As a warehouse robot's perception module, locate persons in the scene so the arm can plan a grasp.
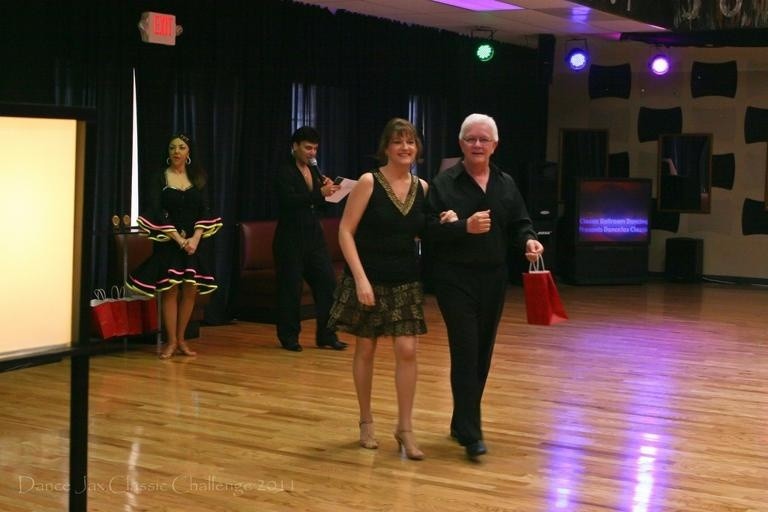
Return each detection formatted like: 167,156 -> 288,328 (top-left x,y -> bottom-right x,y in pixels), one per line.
125,134 -> 226,359
421,114 -> 545,457
326,118 -> 460,460
272,125 -> 350,352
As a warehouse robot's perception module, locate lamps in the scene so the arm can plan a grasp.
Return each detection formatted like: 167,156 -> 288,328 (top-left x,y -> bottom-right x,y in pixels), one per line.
565,38 -> 590,72
472,31 -> 497,63
650,47 -> 671,76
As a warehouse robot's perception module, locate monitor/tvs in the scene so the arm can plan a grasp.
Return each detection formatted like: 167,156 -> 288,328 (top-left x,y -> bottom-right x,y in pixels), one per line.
561,176 -> 652,245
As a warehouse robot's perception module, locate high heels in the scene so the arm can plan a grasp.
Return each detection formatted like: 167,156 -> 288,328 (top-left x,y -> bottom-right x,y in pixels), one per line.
360,415 -> 378,449
395,429 -> 426,459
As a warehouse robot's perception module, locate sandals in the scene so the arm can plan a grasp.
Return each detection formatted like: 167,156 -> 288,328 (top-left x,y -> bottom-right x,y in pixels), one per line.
160,341 -> 197,359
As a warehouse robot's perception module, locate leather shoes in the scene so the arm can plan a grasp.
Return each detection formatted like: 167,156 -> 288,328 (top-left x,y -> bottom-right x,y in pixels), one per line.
282,341 -> 303,352
317,339 -> 349,349
451,426 -> 486,456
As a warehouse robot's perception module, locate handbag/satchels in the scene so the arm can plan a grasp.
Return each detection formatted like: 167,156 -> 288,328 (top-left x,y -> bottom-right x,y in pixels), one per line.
89,285 -> 158,340
522,252 -> 569,326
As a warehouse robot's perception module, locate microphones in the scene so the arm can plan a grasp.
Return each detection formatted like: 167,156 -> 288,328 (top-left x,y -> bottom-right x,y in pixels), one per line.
308,157 -> 324,187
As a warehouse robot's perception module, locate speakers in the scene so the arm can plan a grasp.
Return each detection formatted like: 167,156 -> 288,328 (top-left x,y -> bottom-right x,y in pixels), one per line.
538,34 -> 555,65
666,237 -> 703,283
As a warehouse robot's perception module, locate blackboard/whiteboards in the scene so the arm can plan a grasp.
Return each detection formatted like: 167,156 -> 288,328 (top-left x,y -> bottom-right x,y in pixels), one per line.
0,112 -> 82,365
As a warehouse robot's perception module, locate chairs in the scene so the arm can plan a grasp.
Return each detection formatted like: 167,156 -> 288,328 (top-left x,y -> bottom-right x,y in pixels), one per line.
113,233 -> 212,347
234,217 -> 351,321
